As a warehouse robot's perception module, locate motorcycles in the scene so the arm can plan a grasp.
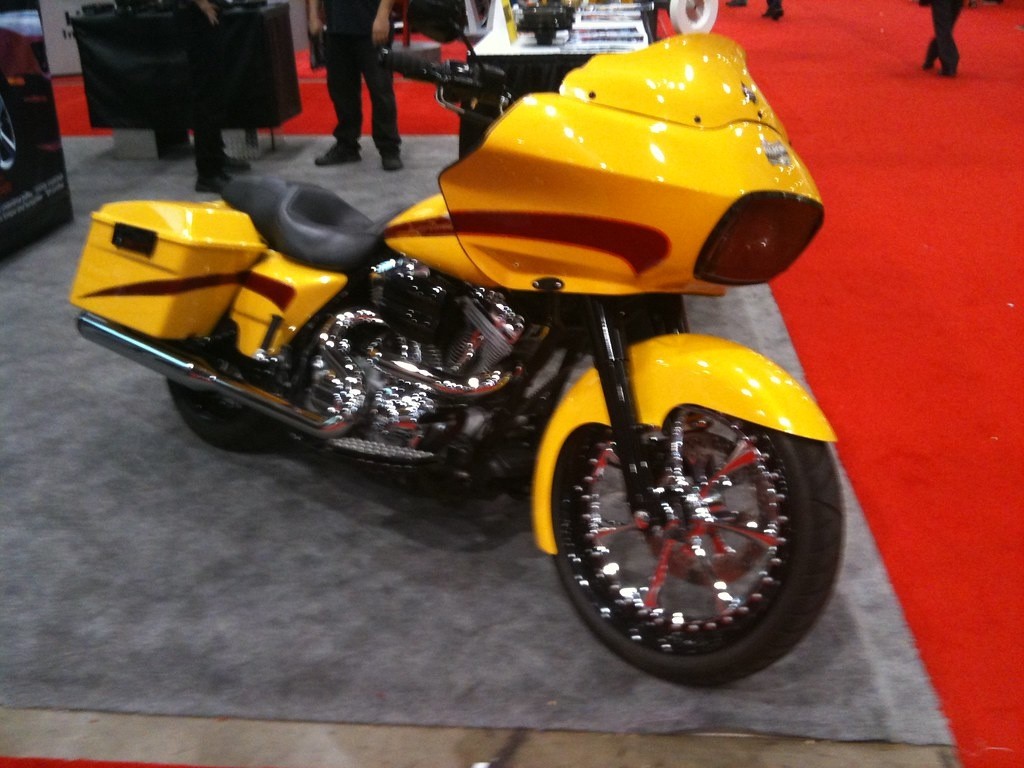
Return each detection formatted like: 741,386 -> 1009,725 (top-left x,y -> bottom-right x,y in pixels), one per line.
62,32 -> 851,691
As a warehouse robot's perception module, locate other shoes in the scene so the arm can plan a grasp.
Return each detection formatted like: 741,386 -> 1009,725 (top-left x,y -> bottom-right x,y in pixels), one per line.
195,172 -> 232,192
771,6 -> 782,19
922,50 -> 936,70
218,154 -> 249,171
380,151 -> 402,169
939,64 -> 956,75
315,146 -> 363,166
726,0 -> 746,6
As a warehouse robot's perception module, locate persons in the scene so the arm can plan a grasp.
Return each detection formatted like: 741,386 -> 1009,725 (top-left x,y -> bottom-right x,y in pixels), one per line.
155,0 -> 405,193
727,0 -> 784,20
919,0 -> 977,76
